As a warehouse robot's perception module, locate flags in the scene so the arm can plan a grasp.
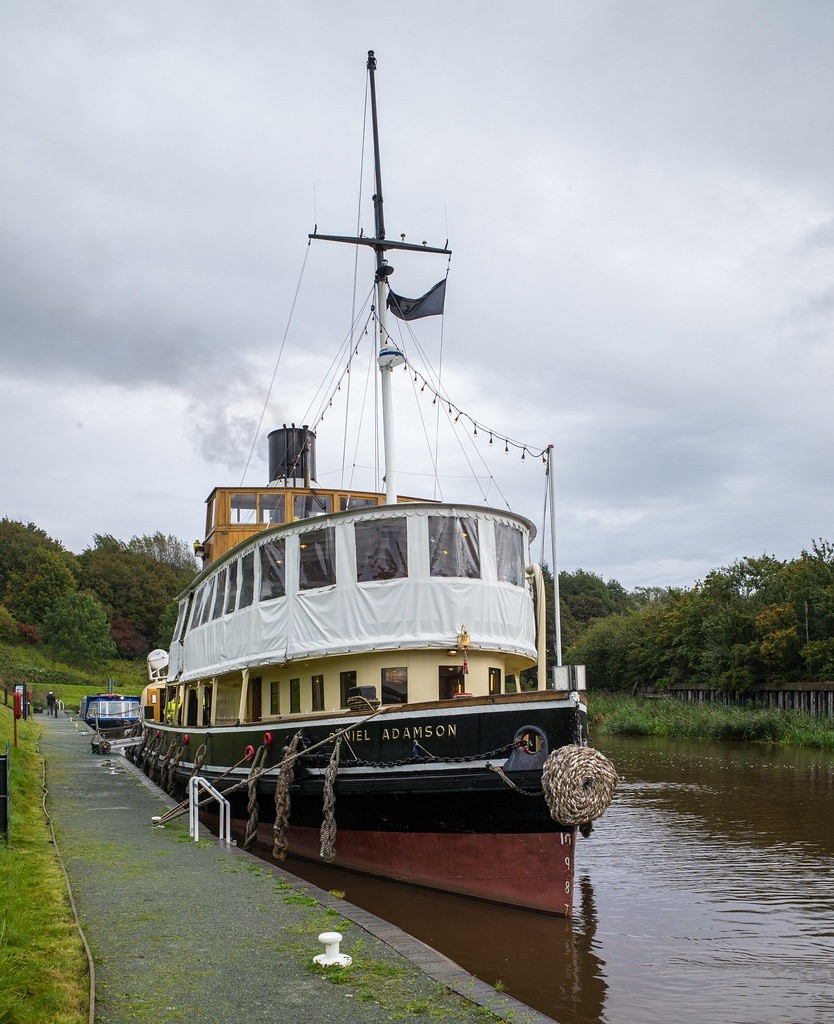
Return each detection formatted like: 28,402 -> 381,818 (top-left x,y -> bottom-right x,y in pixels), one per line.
386,279 -> 445,320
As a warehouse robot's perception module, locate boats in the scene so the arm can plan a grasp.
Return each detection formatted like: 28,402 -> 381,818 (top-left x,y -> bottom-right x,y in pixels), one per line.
76,677 -> 141,732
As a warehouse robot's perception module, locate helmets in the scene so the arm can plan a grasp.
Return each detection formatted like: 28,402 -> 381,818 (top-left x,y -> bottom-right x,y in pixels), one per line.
49,692 -> 53,694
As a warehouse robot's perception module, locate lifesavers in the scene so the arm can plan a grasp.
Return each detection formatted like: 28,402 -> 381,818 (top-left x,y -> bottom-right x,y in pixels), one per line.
245,744 -> 255,762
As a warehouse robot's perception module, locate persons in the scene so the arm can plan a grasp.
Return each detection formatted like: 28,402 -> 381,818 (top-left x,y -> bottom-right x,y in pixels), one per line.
162,693 -> 183,727
46,692 -> 55,715
178,689 -> 208,727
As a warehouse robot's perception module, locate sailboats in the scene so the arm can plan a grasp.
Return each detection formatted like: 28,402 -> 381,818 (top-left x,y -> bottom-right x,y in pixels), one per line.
124,45 -> 626,922
